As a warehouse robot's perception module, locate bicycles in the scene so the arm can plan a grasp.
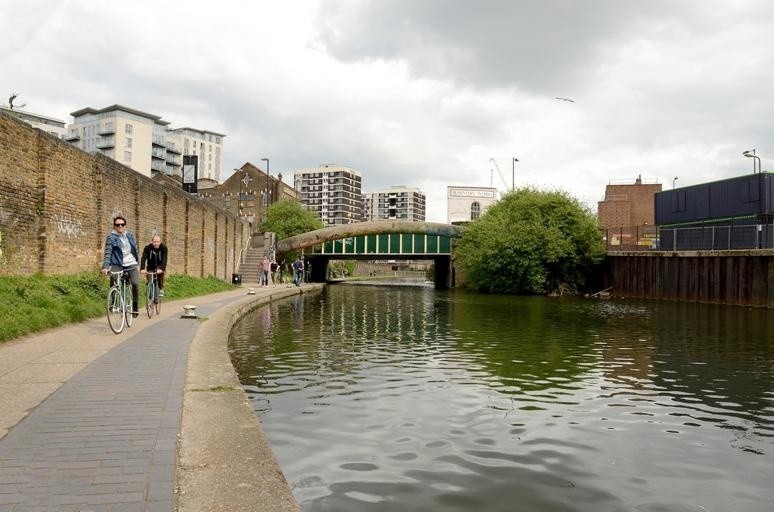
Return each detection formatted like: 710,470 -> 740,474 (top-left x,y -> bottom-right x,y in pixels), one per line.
100,267 -> 139,334
145,270 -> 164,319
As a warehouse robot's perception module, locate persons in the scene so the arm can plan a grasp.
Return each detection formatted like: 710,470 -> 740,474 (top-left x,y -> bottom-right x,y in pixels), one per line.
140,235 -> 168,297
260,255 -> 312,288
101,216 -> 139,318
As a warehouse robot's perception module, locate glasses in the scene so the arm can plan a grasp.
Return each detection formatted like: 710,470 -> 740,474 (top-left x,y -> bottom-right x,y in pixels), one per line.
114,223 -> 126,227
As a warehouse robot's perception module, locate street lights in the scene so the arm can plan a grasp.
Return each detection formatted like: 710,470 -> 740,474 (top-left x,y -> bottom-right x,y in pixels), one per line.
672,177 -> 679,189
513,157 -> 519,191
742,148 -> 762,175
233,168 -> 242,215
260,159 -> 269,206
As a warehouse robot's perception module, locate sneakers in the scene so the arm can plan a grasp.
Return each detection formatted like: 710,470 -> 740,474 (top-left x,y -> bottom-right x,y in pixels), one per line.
109,301 -> 117,312
132,306 -> 139,318
158,290 -> 164,297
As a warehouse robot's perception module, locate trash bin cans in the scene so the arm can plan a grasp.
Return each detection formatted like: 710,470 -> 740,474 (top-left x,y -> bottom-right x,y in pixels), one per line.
232,273 -> 241,284
